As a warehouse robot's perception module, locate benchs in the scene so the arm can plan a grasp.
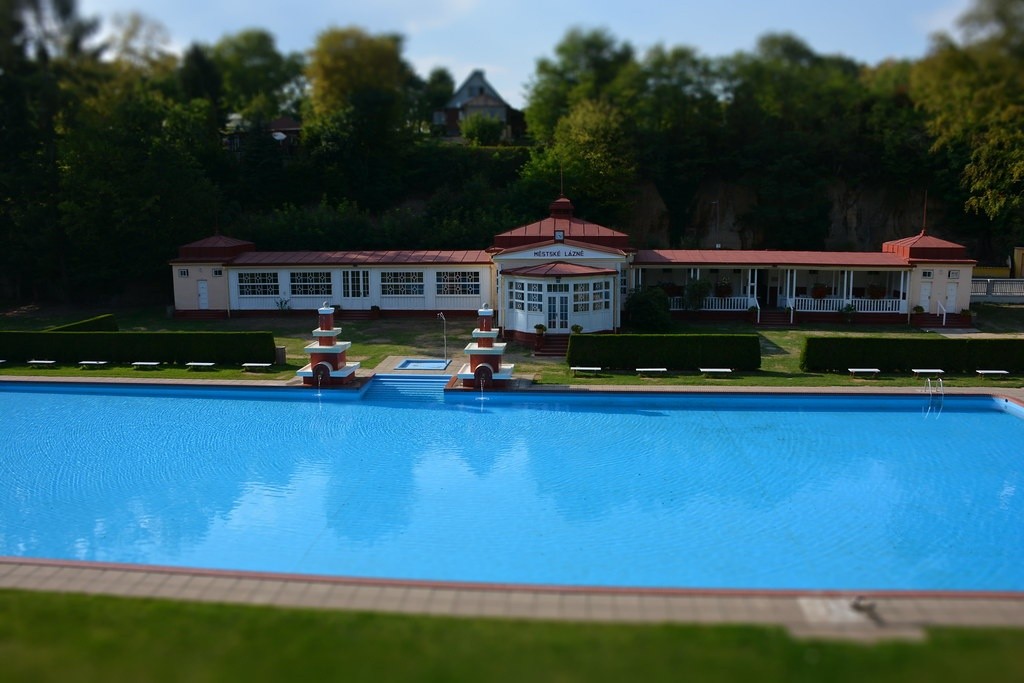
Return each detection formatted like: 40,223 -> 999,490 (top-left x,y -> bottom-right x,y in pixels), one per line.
699,368 -> 732,376
635,367 -> 667,377
78,360 -> 107,368
912,368 -> 944,377
241,362 -> 271,371
848,368 -> 880,377
184,362 -> 215,370
26,360 -> 56,367
570,366 -> 601,375
976,369 -> 1009,378
131,361 -> 160,368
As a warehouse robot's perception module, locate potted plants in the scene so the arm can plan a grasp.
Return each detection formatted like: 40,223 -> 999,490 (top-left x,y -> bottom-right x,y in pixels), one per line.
912,305 -> 924,313
533,323 -> 547,333
570,324 -> 582,333
959,308 -> 969,321
